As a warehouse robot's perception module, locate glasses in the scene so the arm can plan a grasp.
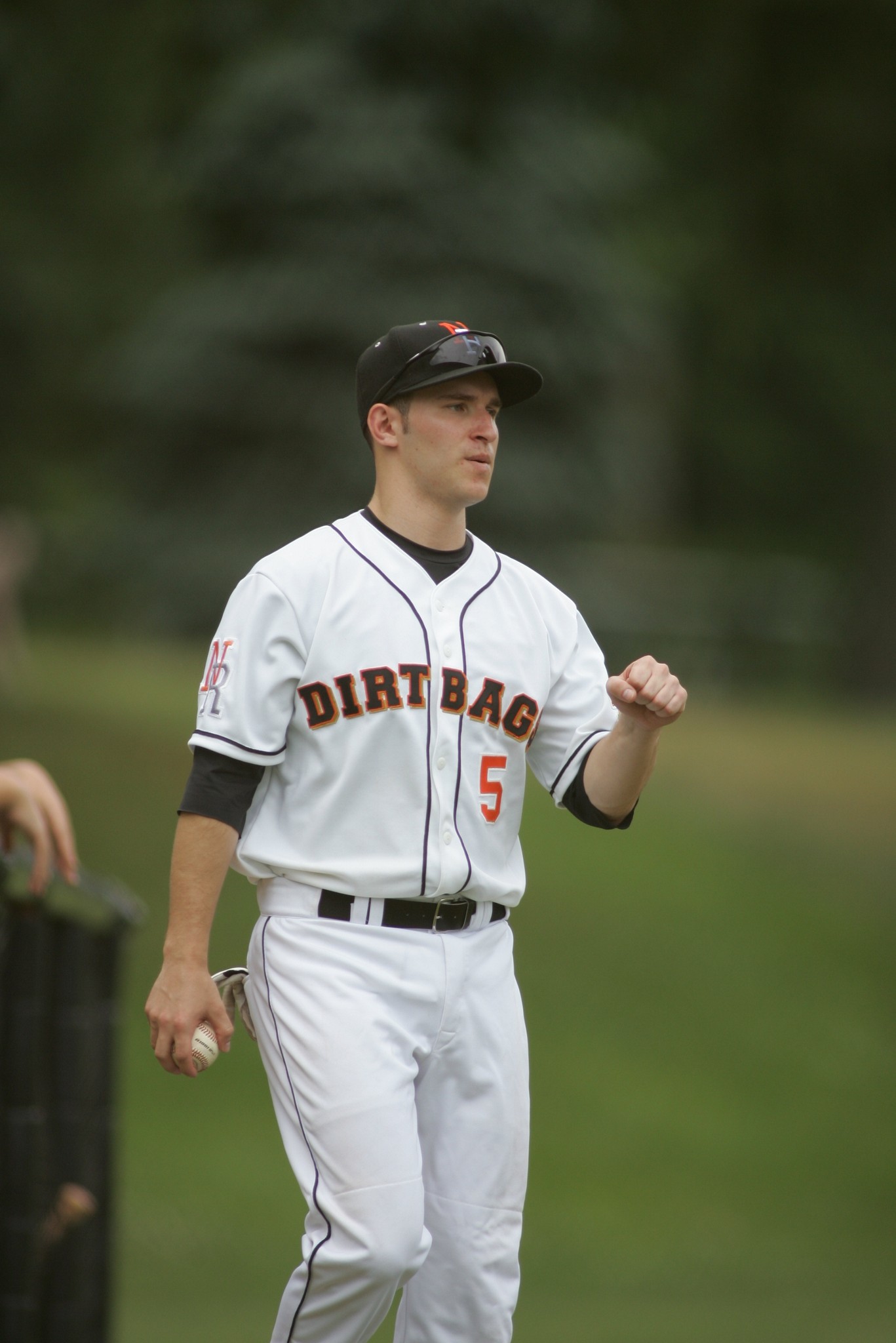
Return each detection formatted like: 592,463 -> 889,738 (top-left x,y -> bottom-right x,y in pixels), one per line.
372,331 -> 508,401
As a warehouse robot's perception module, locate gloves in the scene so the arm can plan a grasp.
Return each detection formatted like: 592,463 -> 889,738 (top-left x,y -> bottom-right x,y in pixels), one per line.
210,964 -> 258,1040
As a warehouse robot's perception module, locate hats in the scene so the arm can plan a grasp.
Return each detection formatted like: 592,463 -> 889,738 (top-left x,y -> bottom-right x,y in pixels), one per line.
355,317 -> 545,427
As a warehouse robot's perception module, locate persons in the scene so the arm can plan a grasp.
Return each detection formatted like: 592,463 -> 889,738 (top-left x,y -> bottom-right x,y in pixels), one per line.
0,759 -> 80,898
141,320 -> 688,1343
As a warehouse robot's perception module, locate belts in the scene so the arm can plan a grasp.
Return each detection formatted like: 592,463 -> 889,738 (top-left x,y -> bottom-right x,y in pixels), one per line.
319,886 -> 506,934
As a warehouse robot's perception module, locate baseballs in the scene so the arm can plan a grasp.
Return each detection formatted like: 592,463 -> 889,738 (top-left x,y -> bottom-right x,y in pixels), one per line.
169,1020 -> 220,1072
